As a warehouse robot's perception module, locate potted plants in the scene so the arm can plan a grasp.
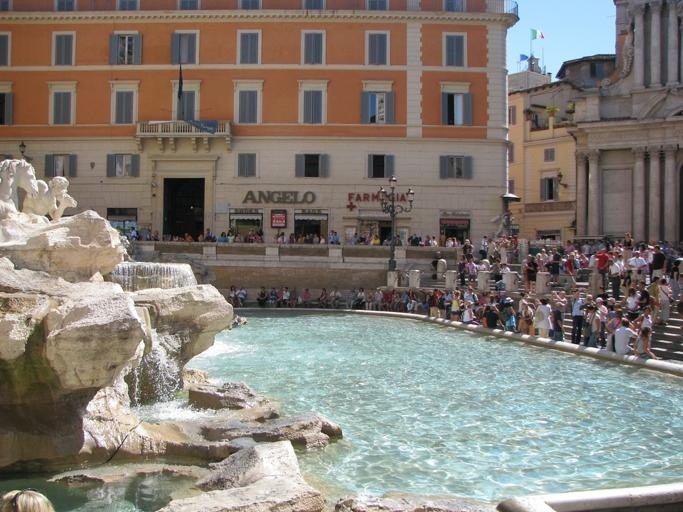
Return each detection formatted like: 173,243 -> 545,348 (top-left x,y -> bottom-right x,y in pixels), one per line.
523,108 -> 534,121
565,103 -> 575,114
545,105 -> 560,118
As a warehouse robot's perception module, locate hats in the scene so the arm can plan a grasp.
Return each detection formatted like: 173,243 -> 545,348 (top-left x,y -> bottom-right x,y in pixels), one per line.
503,296 -> 514,304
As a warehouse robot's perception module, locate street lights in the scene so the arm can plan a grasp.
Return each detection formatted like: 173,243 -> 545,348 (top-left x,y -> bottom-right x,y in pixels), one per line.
377,175 -> 414,271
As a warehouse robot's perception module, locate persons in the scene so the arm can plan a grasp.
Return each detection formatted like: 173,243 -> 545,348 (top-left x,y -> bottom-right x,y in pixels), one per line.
351,233 -> 402,245
117,226 -> 160,241
408,234 -> 430,246
273,230 -> 340,245
185,234 -> 193,241
223,281 -> 431,317
198,227 -> 264,243
429,225 -> 682,361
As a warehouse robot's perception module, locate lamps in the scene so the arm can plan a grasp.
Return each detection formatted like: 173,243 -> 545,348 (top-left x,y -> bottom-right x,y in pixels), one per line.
19,140 -> 33,160
557,172 -> 567,189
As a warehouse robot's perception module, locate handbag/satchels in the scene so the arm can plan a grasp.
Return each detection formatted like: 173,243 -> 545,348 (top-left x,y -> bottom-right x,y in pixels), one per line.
535,307 -> 544,321
667,295 -> 674,304
582,322 -> 592,338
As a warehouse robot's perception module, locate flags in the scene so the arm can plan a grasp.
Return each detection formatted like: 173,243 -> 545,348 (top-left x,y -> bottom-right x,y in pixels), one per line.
519,55 -> 527,61
177,66 -> 183,100
529,28 -> 544,40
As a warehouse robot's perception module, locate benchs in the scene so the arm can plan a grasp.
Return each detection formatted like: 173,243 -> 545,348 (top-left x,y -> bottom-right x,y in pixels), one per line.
244,299 -> 346,306
